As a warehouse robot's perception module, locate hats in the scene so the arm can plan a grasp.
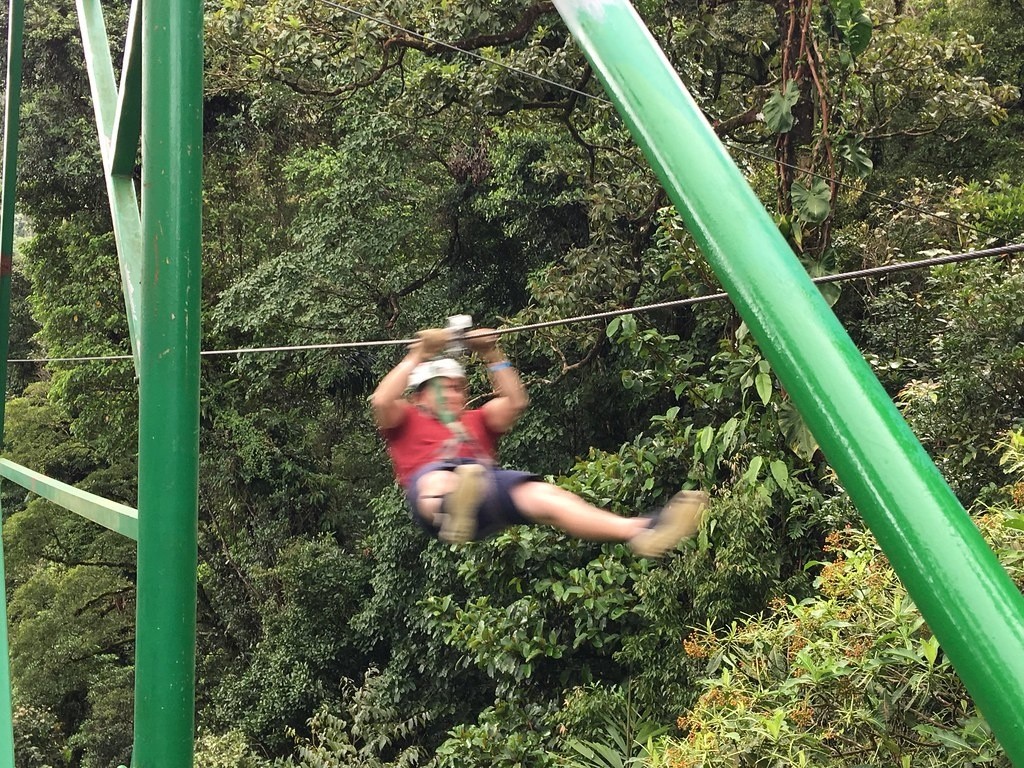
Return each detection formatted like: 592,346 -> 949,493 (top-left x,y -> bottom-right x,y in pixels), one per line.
407,358 -> 466,388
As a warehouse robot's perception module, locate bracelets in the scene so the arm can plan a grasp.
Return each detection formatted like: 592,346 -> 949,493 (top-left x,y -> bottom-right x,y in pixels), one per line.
490,361 -> 513,373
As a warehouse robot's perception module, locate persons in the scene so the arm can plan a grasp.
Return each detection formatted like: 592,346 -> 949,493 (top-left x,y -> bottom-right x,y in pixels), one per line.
373,329 -> 708,556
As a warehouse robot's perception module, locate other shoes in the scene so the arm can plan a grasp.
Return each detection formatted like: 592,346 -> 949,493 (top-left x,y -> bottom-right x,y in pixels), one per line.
630,491 -> 705,554
439,464 -> 487,544
